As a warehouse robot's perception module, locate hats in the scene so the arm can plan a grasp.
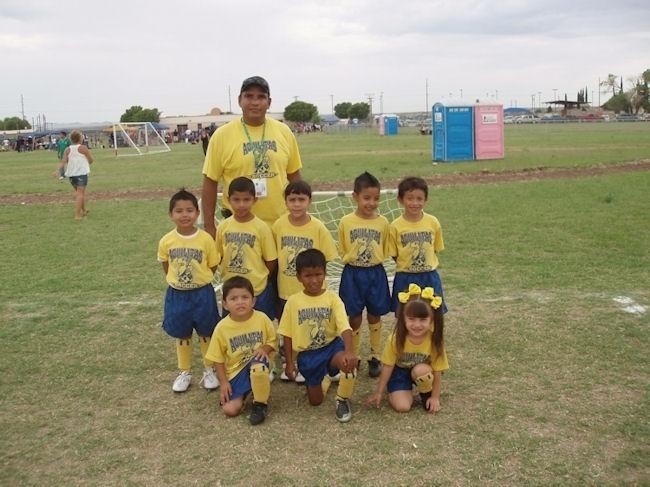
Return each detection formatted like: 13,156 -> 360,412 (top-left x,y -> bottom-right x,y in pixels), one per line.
240,76 -> 269,91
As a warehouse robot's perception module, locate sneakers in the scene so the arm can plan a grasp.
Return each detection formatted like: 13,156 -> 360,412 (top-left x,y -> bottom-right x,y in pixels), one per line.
335,395 -> 351,422
419,391 -> 431,411
249,401 -> 267,425
199,368 -> 218,389
294,371 -> 305,382
172,370 -> 192,392
367,356 -> 381,377
280,362 -> 289,381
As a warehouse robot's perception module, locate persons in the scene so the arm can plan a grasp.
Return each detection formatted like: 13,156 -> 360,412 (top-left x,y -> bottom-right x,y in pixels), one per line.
83,134 -> 89,145
173,129 -> 202,144
277,248 -> 357,422
338,171 -> 390,378
366,283 -> 449,414
215,176 -> 277,383
16,136 -> 33,152
51,130 -> 94,220
390,176 -> 448,317
203,75 -> 303,239
271,180 -> 339,384
56,130 -> 71,180
2,138 -> 10,149
157,190 -> 220,392
200,127 -> 211,156
51,136 -> 56,149
204,275 -> 277,425
